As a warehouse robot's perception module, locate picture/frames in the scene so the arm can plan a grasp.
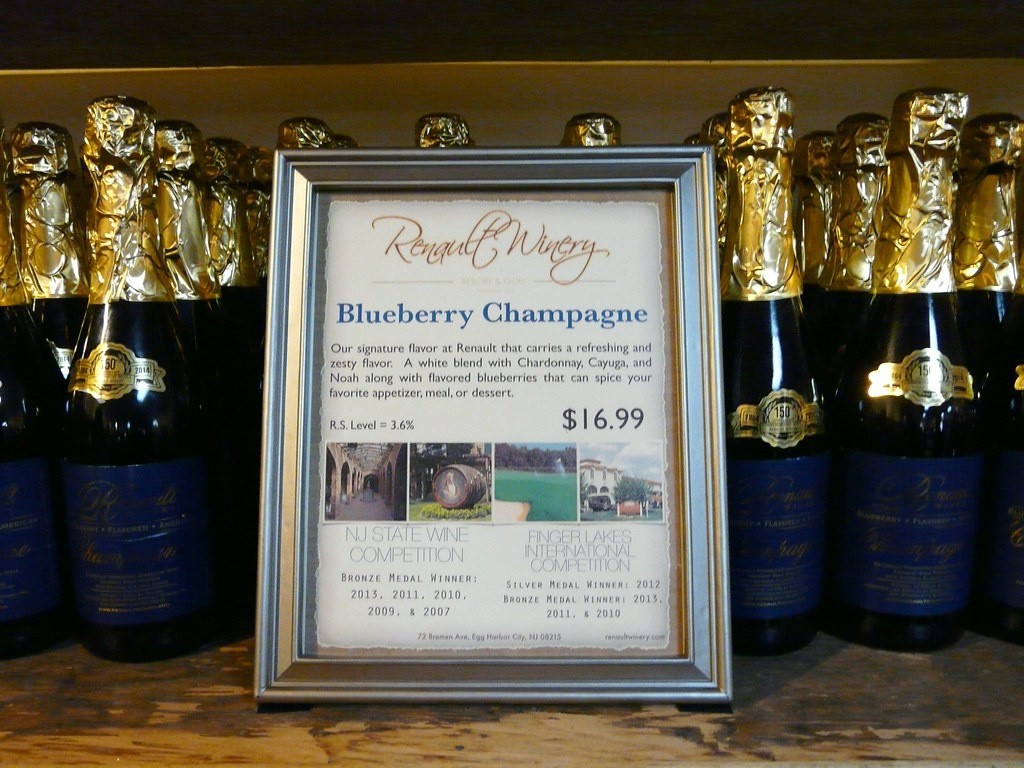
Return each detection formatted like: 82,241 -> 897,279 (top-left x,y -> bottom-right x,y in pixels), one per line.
254,144 -> 748,714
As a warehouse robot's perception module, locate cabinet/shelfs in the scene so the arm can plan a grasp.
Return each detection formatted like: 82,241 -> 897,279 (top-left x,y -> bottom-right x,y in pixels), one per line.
0,0 -> 1024,768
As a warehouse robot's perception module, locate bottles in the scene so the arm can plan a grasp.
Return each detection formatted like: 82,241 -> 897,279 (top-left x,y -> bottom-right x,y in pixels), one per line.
0,85 -> 1024,663
